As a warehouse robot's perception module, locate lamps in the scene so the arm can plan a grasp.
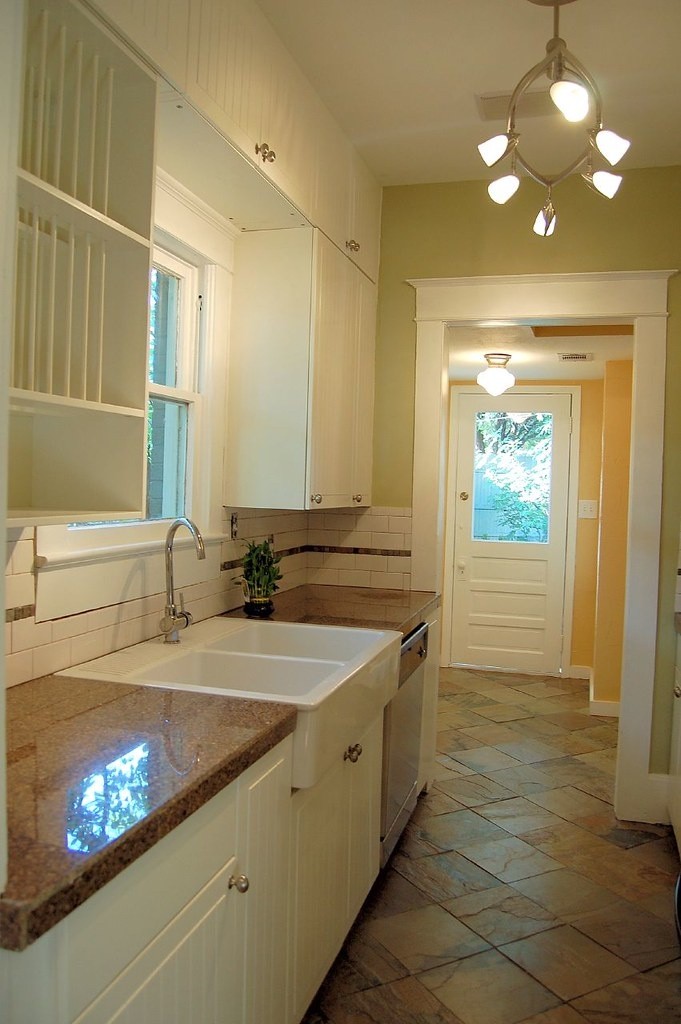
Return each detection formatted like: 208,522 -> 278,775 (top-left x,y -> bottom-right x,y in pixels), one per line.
476,353 -> 516,396
477,0 -> 630,238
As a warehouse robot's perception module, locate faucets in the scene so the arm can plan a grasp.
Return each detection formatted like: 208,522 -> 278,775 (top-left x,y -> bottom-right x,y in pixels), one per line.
158,517 -> 207,645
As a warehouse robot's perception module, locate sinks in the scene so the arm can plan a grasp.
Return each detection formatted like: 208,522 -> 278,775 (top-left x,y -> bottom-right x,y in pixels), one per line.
125,648 -> 358,706
202,620 -> 403,665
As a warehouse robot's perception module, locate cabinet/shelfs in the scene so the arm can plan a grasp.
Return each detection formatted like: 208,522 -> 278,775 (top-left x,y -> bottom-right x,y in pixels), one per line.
0,708 -> 381,1024
0,0 -> 384,528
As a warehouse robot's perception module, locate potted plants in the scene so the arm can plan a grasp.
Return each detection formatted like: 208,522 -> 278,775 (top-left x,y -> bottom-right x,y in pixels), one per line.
232,538 -> 282,621
